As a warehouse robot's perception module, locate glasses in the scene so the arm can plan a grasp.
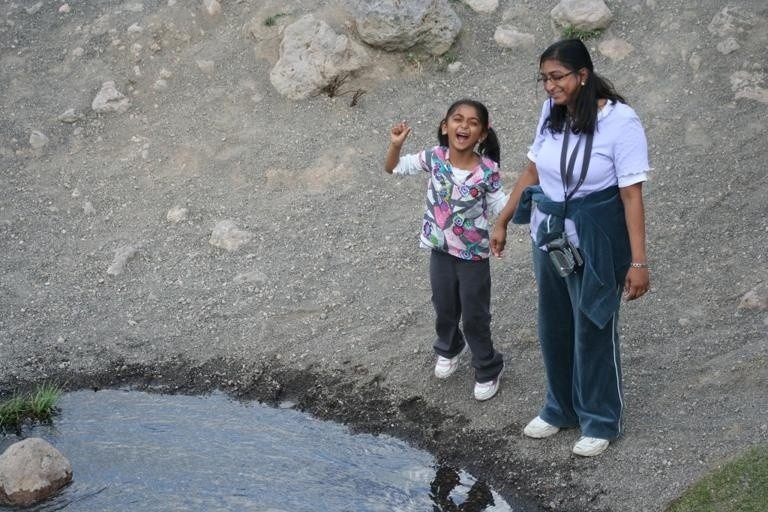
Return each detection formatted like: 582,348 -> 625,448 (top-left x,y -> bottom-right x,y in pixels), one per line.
537,70 -> 574,82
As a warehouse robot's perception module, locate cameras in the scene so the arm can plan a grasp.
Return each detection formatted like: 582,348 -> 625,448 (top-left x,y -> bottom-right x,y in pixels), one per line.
547,238 -> 584,278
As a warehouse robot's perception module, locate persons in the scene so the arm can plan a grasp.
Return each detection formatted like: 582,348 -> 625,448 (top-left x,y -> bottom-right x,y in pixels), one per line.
488,39 -> 653,456
381,98 -> 510,403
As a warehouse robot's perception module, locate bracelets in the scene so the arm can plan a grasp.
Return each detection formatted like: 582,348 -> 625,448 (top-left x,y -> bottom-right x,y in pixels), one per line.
630,262 -> 649,269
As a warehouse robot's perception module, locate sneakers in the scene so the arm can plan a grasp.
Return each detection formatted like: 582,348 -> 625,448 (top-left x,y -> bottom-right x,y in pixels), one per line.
474,362 -> 505,401
573,436 -> 610,456
523,415 -> 560,438
435,343 -> 470,378
